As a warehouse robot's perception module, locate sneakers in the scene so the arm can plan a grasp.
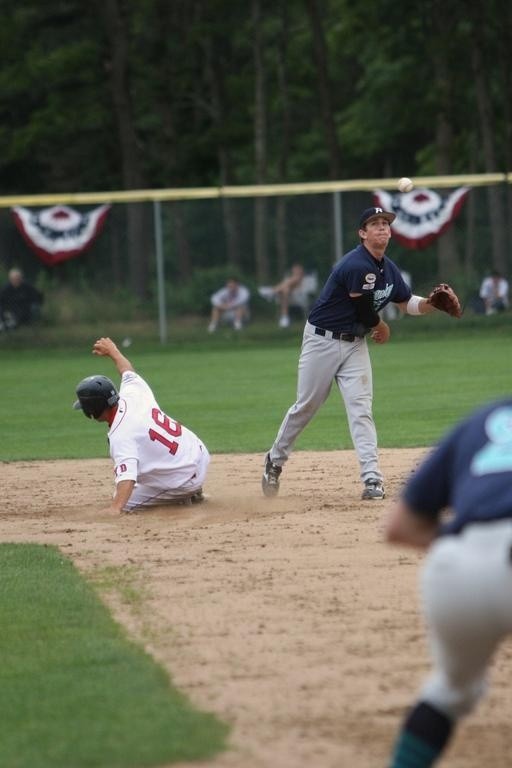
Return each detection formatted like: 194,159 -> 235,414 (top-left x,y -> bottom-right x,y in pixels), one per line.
484,308 -> 497,317
260,449 -> 282,499
207,315 -> 242,335
362,477 -> 385,500
278,315 -> 291,328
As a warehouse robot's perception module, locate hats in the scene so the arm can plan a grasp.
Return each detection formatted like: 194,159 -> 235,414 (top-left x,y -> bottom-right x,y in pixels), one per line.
358,207 -> 397,227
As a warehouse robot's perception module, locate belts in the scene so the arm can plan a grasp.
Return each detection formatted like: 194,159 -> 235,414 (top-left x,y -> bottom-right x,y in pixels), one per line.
314,328 -> 364,342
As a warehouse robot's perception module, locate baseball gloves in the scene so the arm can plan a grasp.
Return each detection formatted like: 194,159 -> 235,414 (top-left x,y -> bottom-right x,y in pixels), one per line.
427,285 -> 461,316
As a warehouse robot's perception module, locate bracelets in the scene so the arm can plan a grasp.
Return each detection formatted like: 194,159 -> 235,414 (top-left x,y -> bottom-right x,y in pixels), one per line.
407,295 -> 425,316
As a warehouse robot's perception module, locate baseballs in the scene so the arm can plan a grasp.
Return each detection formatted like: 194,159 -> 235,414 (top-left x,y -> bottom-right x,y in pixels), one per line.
122,338 -> 131,347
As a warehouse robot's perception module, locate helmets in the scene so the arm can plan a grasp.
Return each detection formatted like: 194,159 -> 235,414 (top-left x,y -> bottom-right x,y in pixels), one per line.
70,374 -> 120,411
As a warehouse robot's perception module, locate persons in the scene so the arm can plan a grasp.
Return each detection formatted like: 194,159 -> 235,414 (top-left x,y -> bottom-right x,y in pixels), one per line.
274,261 -> 315,329
0,267 -> 46,330
378,270 -> 412,320
386,398 -> 512,767
262,205 -> 463,502
206,276 -> 253,331
73,336 -> 210,519
478,271 -> 510,316
